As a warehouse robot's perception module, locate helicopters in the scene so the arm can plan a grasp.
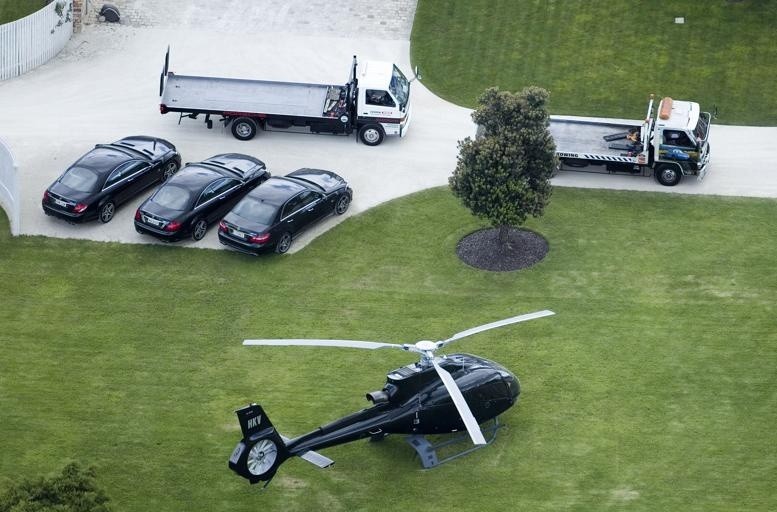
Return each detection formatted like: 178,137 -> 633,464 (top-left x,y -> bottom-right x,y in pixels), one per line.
228,309 -> 559,493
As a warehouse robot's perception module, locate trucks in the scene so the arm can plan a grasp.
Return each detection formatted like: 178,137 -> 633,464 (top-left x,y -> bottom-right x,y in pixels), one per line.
159,41 -> 424,146
547,96 -> 720,186
